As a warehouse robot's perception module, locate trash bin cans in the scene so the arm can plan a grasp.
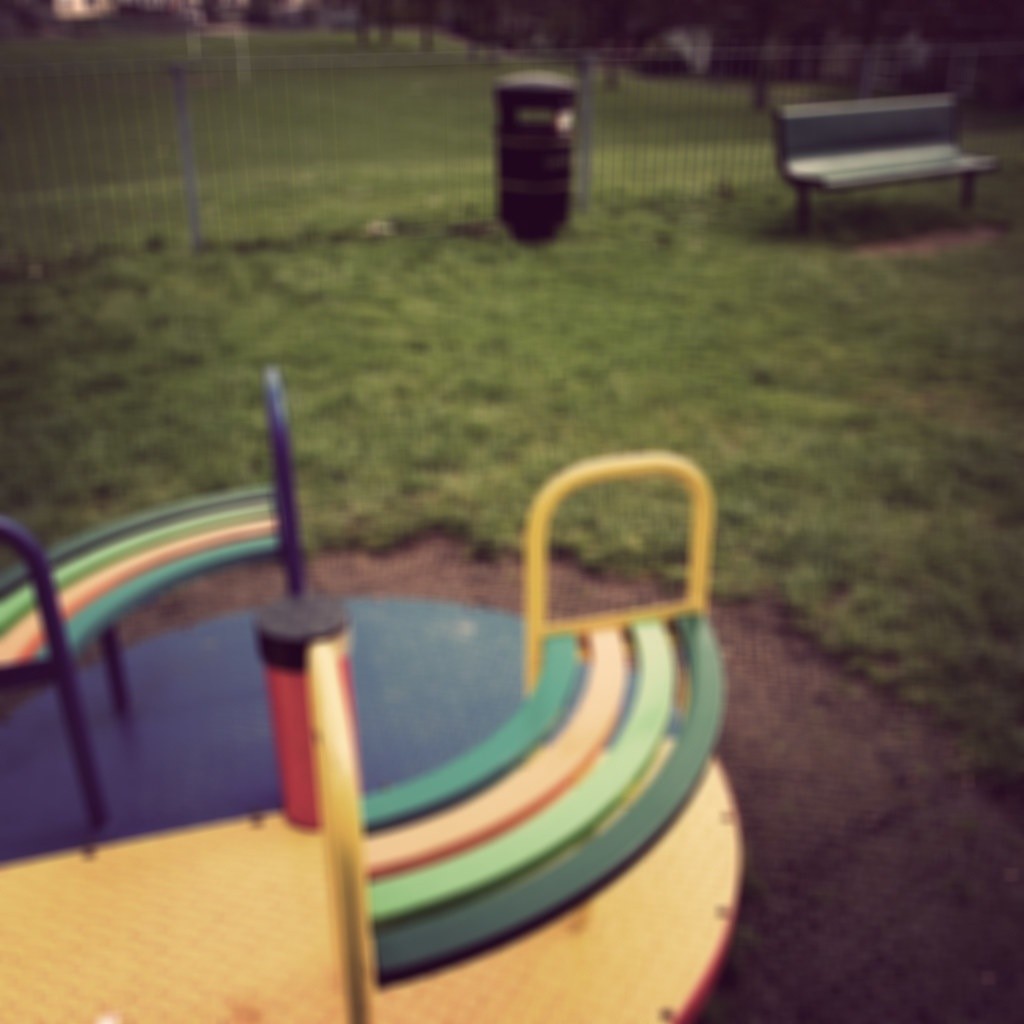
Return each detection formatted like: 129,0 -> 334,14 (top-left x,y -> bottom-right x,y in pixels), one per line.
490,70 -> 580,243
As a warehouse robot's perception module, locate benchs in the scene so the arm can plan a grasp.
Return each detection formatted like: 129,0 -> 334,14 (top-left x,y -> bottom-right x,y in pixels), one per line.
306,453 -> 729,1024
0,370 -> 306,826
773,92 -> 1001,238
